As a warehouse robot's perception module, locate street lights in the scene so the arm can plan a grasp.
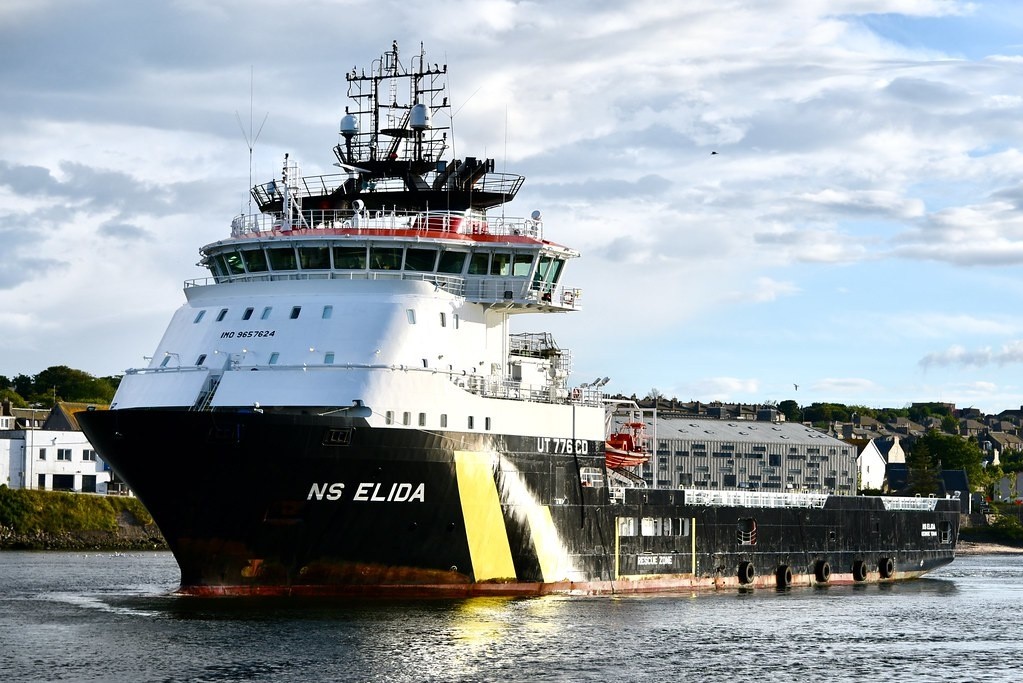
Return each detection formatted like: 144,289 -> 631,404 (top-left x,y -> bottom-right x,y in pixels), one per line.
20,446 -> 24,489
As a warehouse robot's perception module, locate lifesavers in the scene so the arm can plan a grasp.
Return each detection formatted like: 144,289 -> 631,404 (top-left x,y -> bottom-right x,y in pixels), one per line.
573,387 -> 579,397
738,560 -> 754,584
815,560 -> 830,582
563,291 -> 573,304
854,561 -> 868,582
880,558 -> 893,578
776,565 -> 792,587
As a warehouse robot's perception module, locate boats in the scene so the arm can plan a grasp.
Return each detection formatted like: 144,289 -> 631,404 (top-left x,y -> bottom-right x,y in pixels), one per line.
605,433 -> 654,469
71,39 -> 963,603
